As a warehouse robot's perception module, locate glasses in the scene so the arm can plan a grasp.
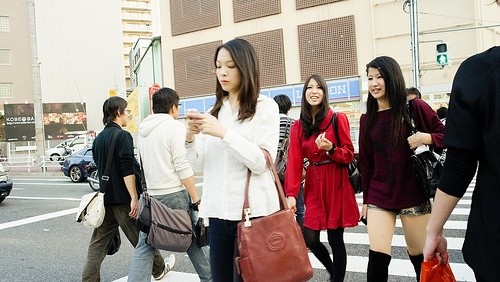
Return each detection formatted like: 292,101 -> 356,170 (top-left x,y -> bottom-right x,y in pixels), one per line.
122,110 -> 132,114
176,103 -> 182,108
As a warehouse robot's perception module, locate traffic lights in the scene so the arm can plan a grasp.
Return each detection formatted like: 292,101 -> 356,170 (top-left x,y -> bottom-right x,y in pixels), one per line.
435,43 -> 448,65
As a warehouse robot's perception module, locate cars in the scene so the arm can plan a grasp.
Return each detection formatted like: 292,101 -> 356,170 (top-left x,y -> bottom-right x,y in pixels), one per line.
44,134 -> 97,184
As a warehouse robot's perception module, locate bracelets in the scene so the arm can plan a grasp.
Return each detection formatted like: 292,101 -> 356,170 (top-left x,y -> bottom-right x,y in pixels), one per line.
185,136 -> 196,144
325,143 -> 336,155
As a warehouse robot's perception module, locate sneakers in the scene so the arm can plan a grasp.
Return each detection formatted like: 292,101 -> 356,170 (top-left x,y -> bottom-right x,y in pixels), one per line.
156,254 -> 176,279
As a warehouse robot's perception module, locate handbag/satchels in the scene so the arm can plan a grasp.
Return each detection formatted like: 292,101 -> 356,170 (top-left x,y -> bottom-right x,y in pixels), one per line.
136,196 -> 152,233
145,198 -> 192,252
74,192 -> 106,229
194,217 -> 206,250
274,118 -> 292,180
419,252 -> 455,282
237,148 -> 314,282
405,100 -> 447,190
332,112 -> 362,193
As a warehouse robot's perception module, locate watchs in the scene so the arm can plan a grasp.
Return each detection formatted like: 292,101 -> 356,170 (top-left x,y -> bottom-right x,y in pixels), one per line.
189,199 -> 201,211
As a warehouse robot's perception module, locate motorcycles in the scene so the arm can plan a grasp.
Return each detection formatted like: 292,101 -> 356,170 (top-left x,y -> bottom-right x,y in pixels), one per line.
87,164 -> 101,192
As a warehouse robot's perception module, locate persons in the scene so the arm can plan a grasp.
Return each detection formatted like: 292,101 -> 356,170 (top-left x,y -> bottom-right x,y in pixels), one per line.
185,37 -> 280,282
436,107 -> 448,126
81,96 -> 176,282
422,45 -> 500,282
273,94 -> 310,251
406,87 -> 422,102
359,56 -> 445,282
284,74 -> 354,282
126,87 -> 213,282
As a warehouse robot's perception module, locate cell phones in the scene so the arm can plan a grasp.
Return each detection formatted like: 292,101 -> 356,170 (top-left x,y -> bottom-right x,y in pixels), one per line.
187,109 -> 199,114
